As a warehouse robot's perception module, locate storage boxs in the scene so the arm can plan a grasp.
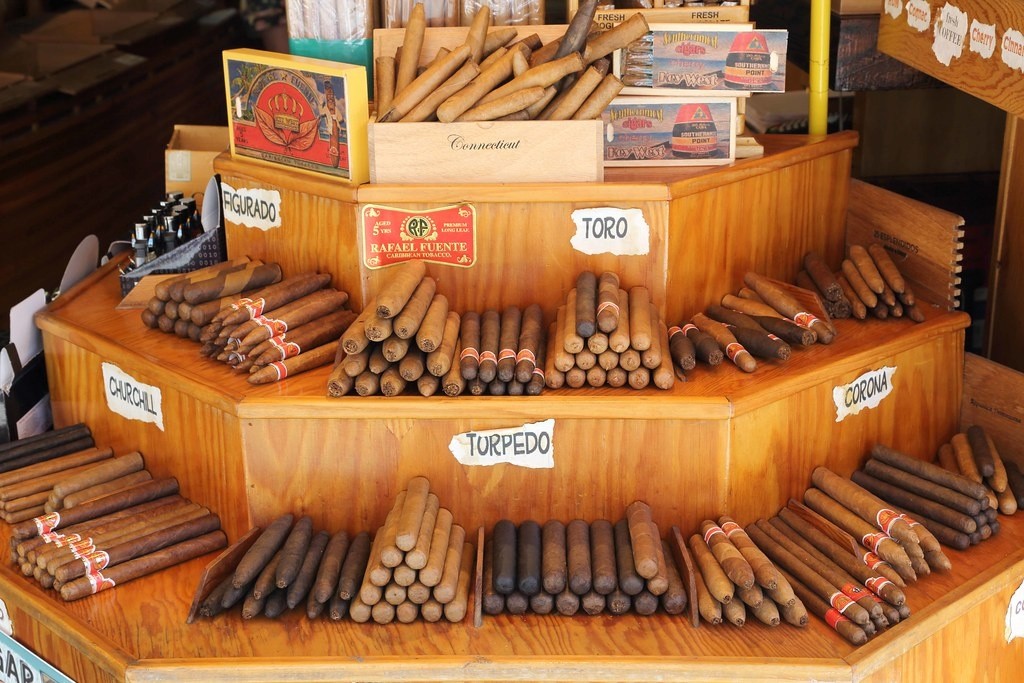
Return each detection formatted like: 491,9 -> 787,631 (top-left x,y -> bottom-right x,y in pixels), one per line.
152,0 -> 786,225
